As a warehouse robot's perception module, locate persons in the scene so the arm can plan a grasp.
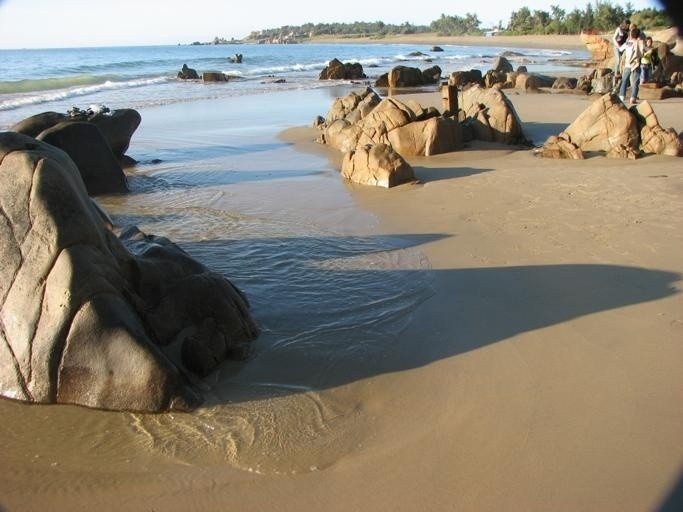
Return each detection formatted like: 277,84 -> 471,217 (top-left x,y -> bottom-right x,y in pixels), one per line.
611,18 -> 629,77
618,28 -> 659,103
639,36 -> 654,83
626,23 -> 646,41
